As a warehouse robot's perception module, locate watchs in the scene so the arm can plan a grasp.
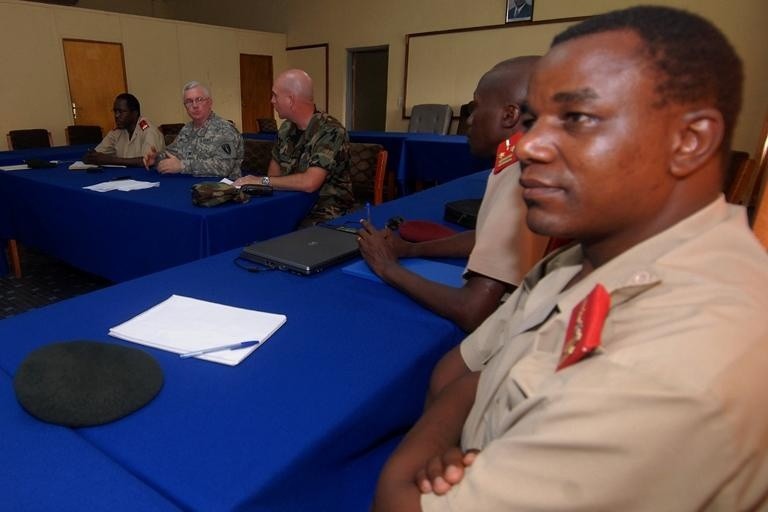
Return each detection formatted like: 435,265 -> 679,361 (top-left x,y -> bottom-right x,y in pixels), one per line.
261,175 -> 270,186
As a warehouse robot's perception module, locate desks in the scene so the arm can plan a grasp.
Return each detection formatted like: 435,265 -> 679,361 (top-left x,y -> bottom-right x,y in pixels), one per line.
242,129 -> 489,201
0,163 -> 494,510
0,150 -> 317,281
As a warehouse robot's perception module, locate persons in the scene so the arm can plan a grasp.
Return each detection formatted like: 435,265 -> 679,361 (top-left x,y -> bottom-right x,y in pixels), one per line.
231,69 -> 353,232
356,56 -> 577,337
374,5 -> 768,512
85,93 -> 165,168
142,81 -> 244,181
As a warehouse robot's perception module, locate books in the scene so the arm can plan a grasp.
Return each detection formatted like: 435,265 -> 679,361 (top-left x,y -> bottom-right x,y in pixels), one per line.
68,160 -> 127,171
107,293 -> 288,366
83,179 -> 160,193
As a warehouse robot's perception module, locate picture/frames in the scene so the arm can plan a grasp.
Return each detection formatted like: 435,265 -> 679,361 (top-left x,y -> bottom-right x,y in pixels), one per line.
504,1 -> 535,24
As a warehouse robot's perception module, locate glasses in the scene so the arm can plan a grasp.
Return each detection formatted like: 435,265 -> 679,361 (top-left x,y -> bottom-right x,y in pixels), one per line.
184,96 -> 208,105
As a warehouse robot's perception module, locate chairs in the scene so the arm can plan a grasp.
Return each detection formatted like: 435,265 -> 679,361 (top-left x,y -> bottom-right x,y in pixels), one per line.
256,118 -> 277,132
243,139 -> 274,176
408,104 -> 452,136
160,122 -> 184,135
345,142 -> 389,207
68,125 -> 102,145
8,129 -> 51,151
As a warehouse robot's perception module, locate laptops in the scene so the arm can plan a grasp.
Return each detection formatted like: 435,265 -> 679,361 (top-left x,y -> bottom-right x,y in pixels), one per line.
241,227 -> 360,275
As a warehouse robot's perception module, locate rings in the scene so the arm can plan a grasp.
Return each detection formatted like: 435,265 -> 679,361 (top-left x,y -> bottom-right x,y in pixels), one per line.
356,237 -> 361,241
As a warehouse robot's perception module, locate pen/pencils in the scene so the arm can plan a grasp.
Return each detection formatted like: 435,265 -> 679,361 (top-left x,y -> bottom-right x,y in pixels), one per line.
366,202 -> 372,224
179,339 -> 260,360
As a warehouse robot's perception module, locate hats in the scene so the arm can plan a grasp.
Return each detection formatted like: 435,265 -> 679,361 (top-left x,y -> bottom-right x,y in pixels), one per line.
192,182 -> 250,207
